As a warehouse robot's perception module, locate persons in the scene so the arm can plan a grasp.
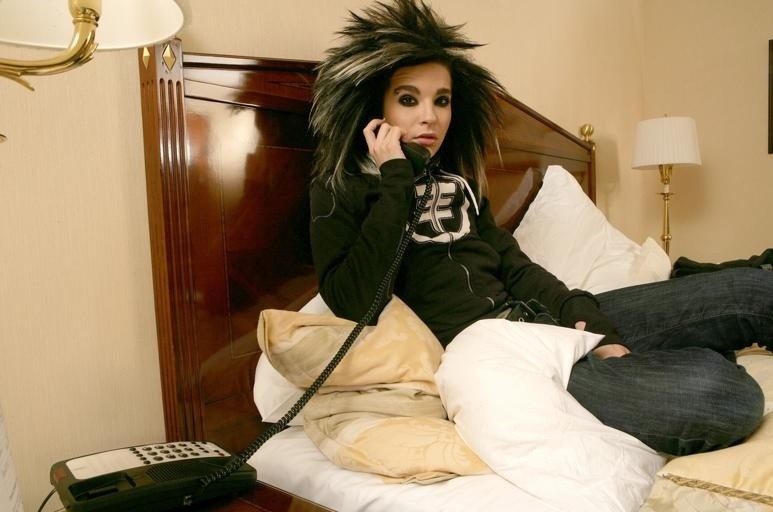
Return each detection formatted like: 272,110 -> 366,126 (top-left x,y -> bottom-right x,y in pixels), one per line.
307,0 -> 773,456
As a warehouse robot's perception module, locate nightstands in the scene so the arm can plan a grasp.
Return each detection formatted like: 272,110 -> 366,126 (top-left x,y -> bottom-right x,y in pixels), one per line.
59,477 -> 334,512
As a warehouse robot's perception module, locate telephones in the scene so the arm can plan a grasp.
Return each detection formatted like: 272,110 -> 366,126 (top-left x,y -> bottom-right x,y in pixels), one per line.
366,110 -> 431,177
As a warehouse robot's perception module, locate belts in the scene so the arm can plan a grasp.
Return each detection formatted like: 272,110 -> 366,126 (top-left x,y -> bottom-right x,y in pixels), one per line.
505,299 -> 547,321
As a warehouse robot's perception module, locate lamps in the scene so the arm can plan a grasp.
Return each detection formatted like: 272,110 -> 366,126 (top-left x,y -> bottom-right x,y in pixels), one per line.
0,1 -> 187,98
627,112 -> 704,261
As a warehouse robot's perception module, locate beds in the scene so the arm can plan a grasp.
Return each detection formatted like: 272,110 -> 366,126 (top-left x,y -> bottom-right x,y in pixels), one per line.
133,33 -> 771,510
